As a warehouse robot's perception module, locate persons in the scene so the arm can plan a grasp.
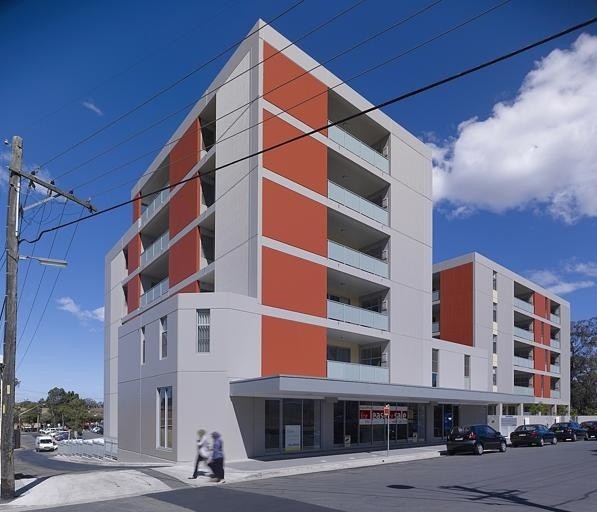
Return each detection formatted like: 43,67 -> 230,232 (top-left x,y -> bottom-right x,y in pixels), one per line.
189,430 -> 211,479
210,431 -> 225,483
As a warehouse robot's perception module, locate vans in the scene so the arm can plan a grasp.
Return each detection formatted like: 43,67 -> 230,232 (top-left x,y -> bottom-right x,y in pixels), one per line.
34,435 -> 54,452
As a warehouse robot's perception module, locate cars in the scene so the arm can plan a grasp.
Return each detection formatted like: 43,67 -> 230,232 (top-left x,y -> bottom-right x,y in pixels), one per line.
39,425 -> 71,440
509,423 -> 557,446
53,443 -> 58,450
446,424 -> 507,455
579,420 -> 596,437
548,421 -> 587,438
91,427 -> 103,434
